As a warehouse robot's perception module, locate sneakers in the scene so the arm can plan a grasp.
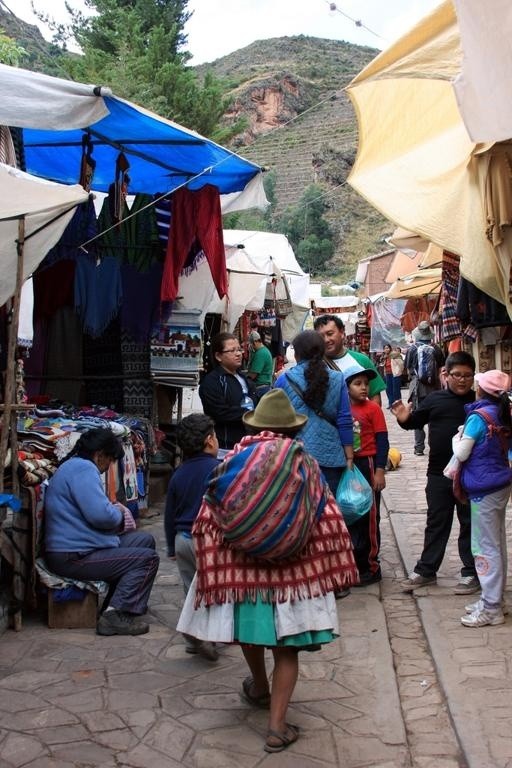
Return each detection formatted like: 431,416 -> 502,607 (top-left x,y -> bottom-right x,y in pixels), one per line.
94,606 -> 152,637
453,574 -> 484,595
413,450 -> 425,457
180,631 -> 220,662
400,570 -> 439,589
459,597 -> 508,628
334,577 -> 382,601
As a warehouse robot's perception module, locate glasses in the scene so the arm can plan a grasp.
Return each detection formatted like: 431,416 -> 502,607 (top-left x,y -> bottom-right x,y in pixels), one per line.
218,348 -> 245,355
448,370 -> 475,381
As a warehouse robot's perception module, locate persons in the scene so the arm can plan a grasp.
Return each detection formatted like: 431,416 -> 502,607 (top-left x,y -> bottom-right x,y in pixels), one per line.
448,367 -> 512,628
387,347 -> 481,596
391,348 -> 407,403
244,329 -> 274,402
314,314 -> 386,413
379,343 -> 404,410
162,411 -> 222,660
268,330 -> 355,599
404,320 -> 442,457
198,333 -> 260,460
41,426 -> 161,637
171,386 -> 361,756
341,364 -> 388,588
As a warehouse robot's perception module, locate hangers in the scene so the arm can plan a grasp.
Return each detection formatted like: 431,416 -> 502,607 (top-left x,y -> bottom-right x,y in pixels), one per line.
159,176 -> 181,199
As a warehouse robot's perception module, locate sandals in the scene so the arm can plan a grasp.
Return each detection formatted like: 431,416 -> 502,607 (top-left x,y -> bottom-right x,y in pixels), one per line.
241,675 -> 272,711
262,722 -> 300,754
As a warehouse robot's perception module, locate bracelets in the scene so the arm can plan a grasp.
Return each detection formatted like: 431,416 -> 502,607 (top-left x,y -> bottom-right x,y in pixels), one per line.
346,456 -> 353,460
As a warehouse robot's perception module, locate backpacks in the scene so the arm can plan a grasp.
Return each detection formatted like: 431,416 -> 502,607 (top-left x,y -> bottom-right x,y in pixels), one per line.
413,340 -> 438,386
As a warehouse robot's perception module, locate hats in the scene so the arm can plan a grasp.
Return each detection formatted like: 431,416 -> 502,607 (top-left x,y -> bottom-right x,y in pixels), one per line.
241,387 -> 310,433
411,320 -> 437,342
473,368 -> 511,399
341,365 -> 379,383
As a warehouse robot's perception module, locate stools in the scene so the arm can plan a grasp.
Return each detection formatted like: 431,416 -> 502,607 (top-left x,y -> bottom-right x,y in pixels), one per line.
47,586 -> 97,629
